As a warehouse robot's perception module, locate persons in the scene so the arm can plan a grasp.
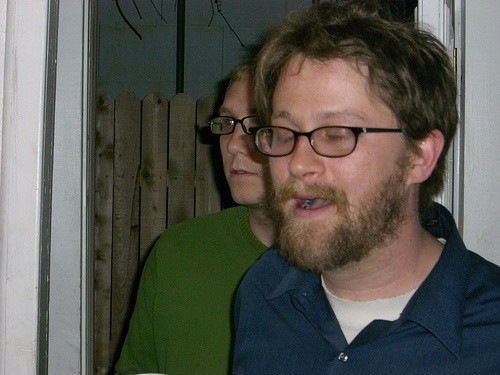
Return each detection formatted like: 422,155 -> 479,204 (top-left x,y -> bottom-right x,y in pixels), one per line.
230,0 -> 500,375
112,29 -> 278,375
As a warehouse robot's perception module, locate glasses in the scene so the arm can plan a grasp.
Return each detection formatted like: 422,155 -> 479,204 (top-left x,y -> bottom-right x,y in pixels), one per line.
209,115 -> 262,135
248,126 -> 404,158
